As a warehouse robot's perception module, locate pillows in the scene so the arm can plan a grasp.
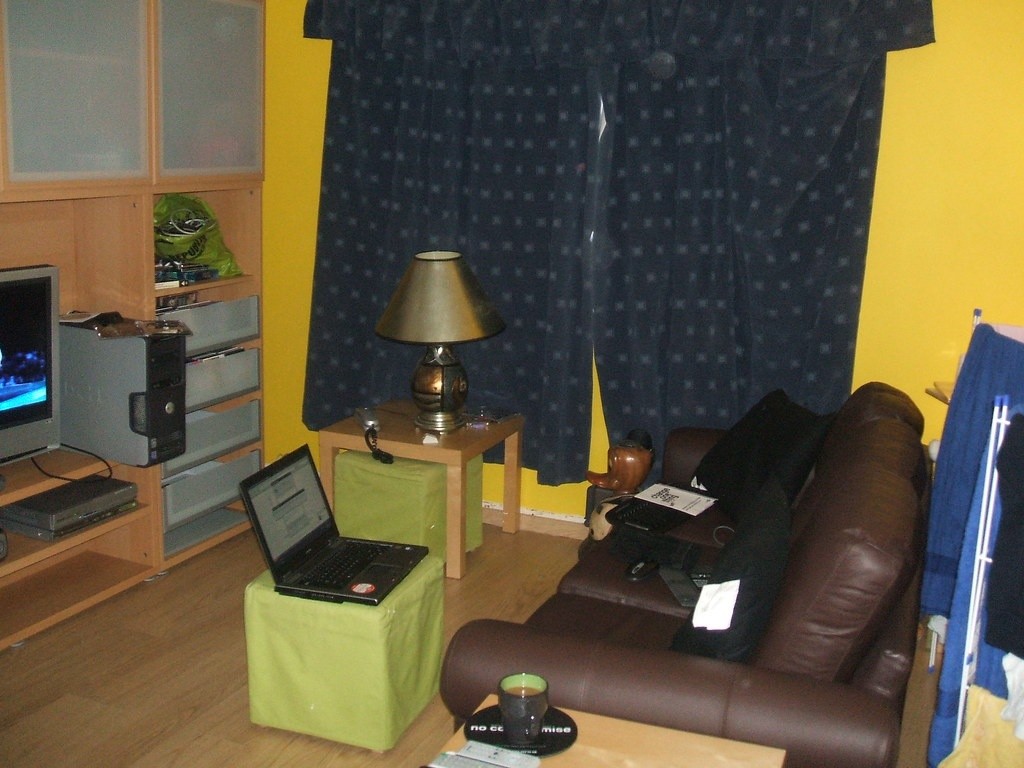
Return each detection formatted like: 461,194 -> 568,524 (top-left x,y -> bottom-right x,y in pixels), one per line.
690,388 -> 839,526
667,470 -> 794,664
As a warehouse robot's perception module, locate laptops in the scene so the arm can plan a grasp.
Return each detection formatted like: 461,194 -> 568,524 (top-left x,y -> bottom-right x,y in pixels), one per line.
238,442 -> 430,607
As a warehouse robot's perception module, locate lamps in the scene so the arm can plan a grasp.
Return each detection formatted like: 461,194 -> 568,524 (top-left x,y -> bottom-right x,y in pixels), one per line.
372,249 -> 508,436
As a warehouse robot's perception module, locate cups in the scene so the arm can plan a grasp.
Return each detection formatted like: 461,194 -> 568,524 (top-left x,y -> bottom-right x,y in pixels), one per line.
356,407 -> 381,432
498,673 -> 549,746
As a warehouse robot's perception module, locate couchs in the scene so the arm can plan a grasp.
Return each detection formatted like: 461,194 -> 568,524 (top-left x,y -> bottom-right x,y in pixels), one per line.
439,381 -> 937,768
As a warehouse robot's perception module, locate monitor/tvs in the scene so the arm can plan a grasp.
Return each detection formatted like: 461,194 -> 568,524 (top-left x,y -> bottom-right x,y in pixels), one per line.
0,263 -> 61,493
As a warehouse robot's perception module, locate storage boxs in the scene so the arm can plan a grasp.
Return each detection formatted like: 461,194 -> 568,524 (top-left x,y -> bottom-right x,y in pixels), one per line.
184,349 -> 259,415
159,400 -> 261,479
162,451 -> 261,535
155,296 -> 259,358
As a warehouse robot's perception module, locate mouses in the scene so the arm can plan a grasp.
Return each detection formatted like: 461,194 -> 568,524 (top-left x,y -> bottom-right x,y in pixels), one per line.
624,556 -> 660,584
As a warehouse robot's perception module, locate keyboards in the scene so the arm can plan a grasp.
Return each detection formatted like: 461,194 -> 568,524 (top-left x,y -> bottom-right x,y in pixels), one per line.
604,482 -> 704,536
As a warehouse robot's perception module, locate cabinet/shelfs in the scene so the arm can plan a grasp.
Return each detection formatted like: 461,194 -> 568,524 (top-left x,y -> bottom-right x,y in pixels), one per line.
0,458 -> 163,653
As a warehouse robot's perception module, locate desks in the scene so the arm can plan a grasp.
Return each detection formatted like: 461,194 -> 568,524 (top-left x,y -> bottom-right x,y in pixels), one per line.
419,693 -> 787,768
318,400 -> 526,580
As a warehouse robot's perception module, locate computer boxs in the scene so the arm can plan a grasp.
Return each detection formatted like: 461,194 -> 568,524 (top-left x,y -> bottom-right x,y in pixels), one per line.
60,318 -> 186,467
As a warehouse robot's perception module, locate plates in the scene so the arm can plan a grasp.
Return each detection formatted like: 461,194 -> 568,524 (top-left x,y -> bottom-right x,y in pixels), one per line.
463,704 -> 578,756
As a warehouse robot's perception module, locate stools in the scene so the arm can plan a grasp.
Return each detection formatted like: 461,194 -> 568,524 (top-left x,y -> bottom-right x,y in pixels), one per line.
243,555 -> 445,757
333,451 -> 483,563
